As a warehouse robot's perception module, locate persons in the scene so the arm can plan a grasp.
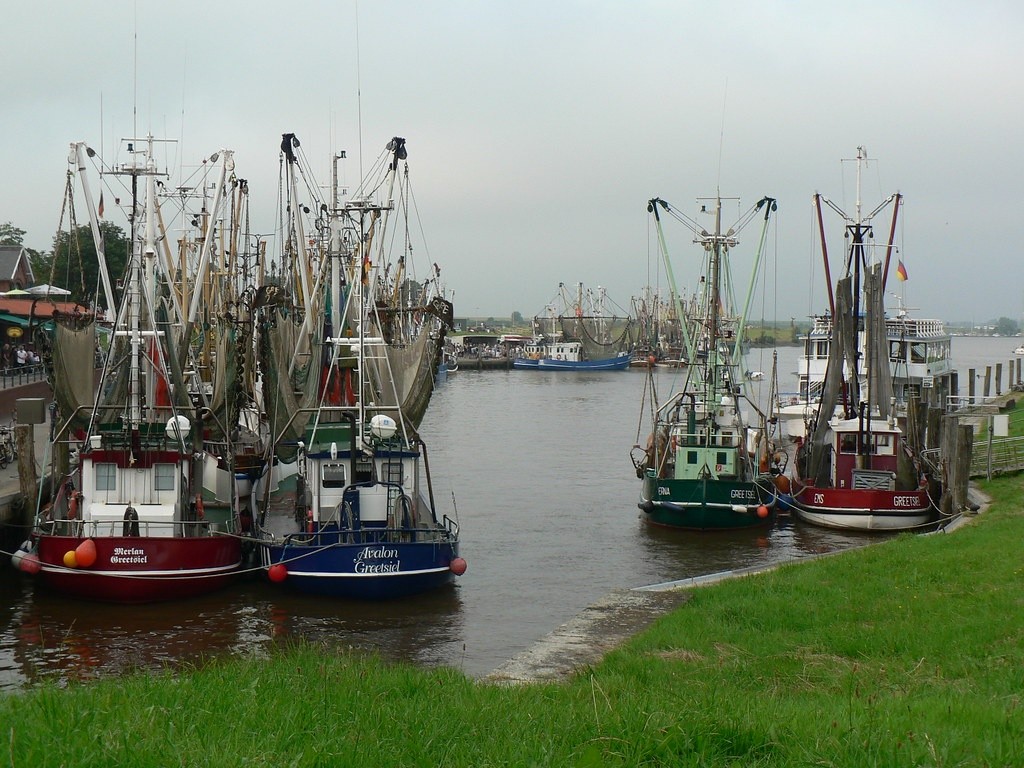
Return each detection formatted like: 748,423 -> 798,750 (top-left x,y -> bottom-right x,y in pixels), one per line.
465,343 -> 522,360
0,344 -> 40,376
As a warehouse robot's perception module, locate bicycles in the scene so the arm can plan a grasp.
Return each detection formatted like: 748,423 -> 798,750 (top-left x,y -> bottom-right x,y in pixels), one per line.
0,418 -> 34,470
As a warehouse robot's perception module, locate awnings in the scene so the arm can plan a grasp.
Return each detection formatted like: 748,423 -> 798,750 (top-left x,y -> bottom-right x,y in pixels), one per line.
27,319 -> 54,330
0,314 -> 38,328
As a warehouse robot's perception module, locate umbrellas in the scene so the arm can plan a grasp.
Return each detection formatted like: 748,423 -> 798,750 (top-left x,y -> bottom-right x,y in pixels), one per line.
26,284 -> 71,301
6,287 -> 31,299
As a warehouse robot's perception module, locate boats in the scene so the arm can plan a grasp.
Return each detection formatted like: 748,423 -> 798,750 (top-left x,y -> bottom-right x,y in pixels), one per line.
441,280 -> 751,374
630,130 -> 791,532
250,29 -> 462,604
772,145 -> 954,530
12,27 -> 266,604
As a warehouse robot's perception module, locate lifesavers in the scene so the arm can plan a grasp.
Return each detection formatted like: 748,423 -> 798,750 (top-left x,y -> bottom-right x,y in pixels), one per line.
557,355 -> 561,359
307,510 -> 313,532
197,493 -> 205,517
70,490 -> 77,518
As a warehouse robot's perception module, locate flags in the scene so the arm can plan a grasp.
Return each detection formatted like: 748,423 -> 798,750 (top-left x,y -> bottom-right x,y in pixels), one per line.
897,260 -> 908,281
